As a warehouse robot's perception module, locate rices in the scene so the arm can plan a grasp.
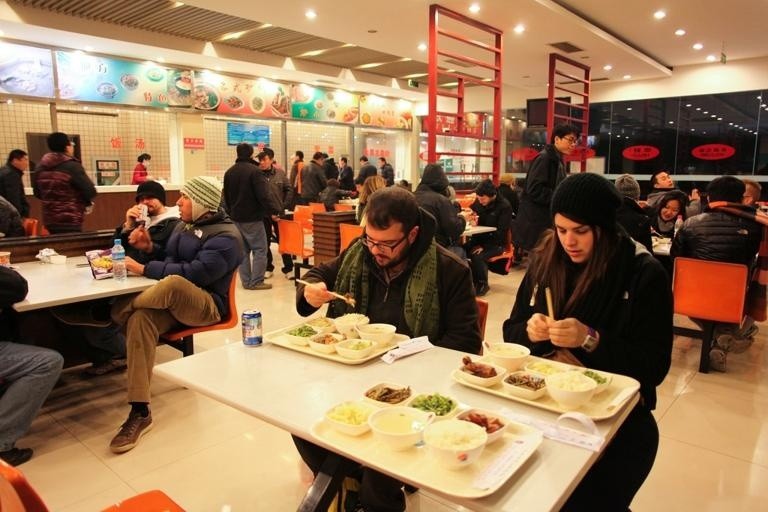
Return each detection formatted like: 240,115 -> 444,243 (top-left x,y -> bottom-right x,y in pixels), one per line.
547,370 -> 593,390
338,312 -> 368,328
432,420 -> 481,449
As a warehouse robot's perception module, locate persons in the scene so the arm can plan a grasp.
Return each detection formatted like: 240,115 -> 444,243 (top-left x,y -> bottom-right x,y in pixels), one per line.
505,172 -> 672,510
0,256 -> 63,465
512,124 -> 585,254
34,133 -> 94,233
221,141 -> 768,369
51,174 -> 244,455
0,151 -> 30,222
1,195 -> 26,238
294,185 -> 484,511
85,179 -> 181,376
131,152 -> 151,183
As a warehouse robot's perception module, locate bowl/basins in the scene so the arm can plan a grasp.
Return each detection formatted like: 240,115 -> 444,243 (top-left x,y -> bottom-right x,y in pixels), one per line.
461,341 -> 612,406
327,387 -> 503,468
288,317 -> 397,358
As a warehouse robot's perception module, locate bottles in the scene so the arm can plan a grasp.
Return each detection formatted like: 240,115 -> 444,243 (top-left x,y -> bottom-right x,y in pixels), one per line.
111,238 -> 126,281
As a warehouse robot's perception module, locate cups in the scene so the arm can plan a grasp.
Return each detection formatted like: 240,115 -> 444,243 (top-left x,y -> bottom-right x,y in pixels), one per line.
0,250 -> 11,268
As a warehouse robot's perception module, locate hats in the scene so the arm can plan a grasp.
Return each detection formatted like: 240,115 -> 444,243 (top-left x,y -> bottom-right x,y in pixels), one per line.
705,176 -> 745,202
551,172 -> 622,219
614,175 -> 640,200
135,180 -> 165,206
476,178 -> 496,192
255,148 -> 273,158
179,176 -> 223,224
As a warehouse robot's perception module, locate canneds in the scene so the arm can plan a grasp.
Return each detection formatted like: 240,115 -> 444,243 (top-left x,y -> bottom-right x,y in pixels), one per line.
241,310 -> 264,346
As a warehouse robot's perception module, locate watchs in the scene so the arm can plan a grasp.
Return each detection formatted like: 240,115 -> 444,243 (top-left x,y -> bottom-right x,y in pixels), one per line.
580,326 -> 598,350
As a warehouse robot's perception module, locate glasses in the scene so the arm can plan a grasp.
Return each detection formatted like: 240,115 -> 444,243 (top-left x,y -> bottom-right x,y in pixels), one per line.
67,140 -> 76,147
360,234 -> 408,255
563,136 -> 577,145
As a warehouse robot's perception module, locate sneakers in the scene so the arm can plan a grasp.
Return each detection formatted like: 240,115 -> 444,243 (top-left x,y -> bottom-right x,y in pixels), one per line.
109,408 -> 154,454
84,359 -> 127,377
263,271 -> 273,279
250,283 -> 272,290
708,347 -> 726,373
340,468 -> 363,512
282,263 -> 293,274
714,330 -> 754,355
49,307 -> 113,328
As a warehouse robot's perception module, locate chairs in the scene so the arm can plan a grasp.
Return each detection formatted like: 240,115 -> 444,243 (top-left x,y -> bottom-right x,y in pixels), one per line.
161,266 -> 239,357
485,226 -> 514,272
275,201 -> 365,286
0,460 -> 186,512
668,256 -> 748,375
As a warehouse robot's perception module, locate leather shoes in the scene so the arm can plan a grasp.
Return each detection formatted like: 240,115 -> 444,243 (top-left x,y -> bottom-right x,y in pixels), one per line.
475,282 -> 489,296
0,448 -> 33,467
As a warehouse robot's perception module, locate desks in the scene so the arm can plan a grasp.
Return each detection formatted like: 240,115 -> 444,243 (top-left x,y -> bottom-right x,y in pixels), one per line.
457,225 -> 497,268
5,255 -> 160,340
